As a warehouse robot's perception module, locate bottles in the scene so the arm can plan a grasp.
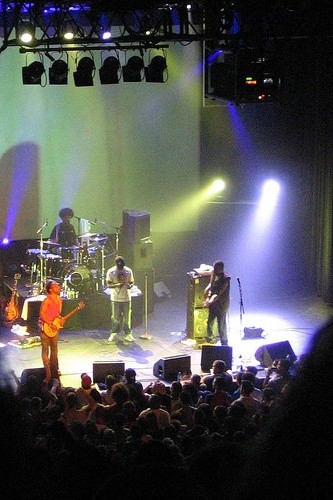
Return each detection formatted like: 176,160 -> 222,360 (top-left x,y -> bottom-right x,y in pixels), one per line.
60,289 -> 63,298
177,372 -> 181,382
67,288 -> 71,299
71,288 -> 76,299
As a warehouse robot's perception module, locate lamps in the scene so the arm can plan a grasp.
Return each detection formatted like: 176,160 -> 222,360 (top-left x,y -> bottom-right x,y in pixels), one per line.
17,0 -> 203,87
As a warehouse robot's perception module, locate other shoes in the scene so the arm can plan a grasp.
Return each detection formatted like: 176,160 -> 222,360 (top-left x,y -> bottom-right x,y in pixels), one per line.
108,333 -> 118,342
197,342 -> 215,349
57,369 -> 61,376
123,334 -> 135,342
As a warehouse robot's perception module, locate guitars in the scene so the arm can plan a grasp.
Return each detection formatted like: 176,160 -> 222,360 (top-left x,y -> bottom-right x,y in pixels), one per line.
42,300 -> 86,338
0,273 -> 22,324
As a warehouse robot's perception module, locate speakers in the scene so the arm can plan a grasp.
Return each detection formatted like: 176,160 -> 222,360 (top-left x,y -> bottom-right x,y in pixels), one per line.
93,362 -> 125,386
124,239 -> 153,271
255,340 -> 297,367
186,275 -> 220,341
201,345 -> 232,373
20,367 -> 46,383
122,208 -> 150,242
152,354 -> 191,382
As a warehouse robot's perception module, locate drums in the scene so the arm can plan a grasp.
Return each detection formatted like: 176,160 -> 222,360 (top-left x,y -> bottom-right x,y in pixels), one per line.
56,246 -> 79,263
58,261 -> 94,293
89,268 -> 101,279
25,248 -> 49,269
36,254 -> 62,276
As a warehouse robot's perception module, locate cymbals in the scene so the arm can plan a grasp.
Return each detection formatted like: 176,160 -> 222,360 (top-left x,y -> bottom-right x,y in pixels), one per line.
36,240 -> 62,246
75,233 -> 99,239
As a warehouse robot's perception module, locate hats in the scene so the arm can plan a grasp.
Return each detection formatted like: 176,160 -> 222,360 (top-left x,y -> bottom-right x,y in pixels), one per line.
81,373 -> 91,387
123,369 -> 136,377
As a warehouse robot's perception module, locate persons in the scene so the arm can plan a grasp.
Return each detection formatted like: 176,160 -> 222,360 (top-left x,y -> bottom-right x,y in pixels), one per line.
46,207 -> 79,256
205,261 -> 231,345
0,318 -> 333,500
106,256 -> 136,340
39,280 -> 64,375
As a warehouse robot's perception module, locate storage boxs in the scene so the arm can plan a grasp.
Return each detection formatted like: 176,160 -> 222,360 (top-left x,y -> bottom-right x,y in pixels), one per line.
117,239 -> 152,269
